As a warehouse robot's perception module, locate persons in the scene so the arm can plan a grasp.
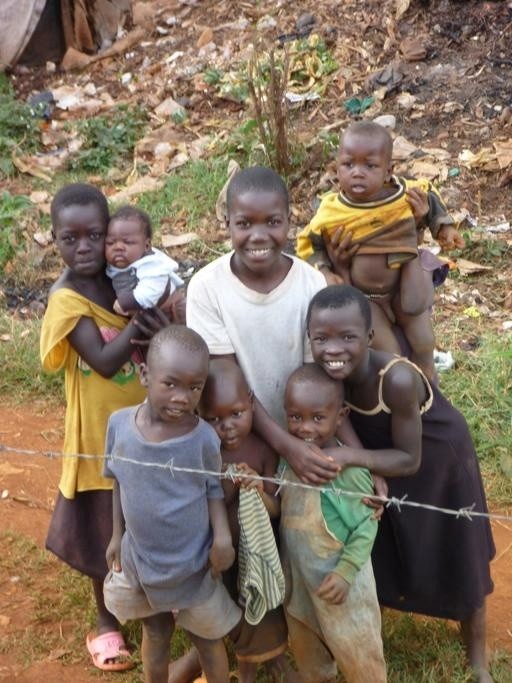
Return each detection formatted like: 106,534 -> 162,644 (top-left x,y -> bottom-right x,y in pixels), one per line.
38,119 -> 496,683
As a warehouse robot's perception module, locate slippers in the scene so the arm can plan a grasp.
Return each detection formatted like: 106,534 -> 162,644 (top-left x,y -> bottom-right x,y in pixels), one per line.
87,630 -> 137,672
